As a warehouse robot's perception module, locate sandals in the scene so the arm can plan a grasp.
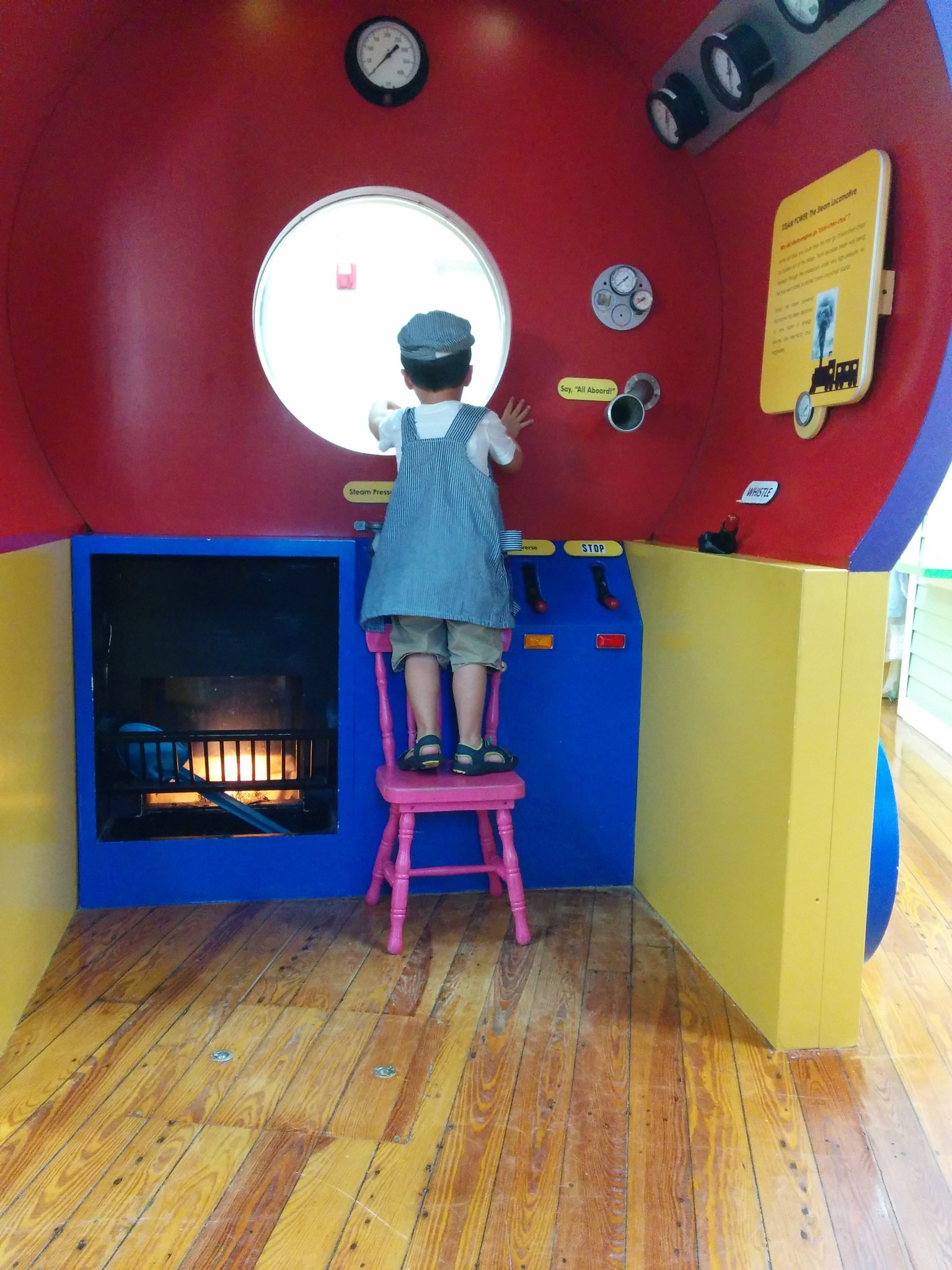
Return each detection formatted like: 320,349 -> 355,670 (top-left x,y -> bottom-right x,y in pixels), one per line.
451,736 -> 519,776
397,732 -> 442,770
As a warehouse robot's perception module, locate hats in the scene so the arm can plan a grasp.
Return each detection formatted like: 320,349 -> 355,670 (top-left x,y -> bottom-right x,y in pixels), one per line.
397,310 -> 475,360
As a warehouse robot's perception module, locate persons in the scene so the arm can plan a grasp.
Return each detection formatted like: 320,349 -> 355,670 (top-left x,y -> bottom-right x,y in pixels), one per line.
357,310 -> 535,777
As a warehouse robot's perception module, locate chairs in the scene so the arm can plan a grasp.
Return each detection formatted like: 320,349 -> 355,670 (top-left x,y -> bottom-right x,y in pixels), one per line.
360,625 -> 536,955
